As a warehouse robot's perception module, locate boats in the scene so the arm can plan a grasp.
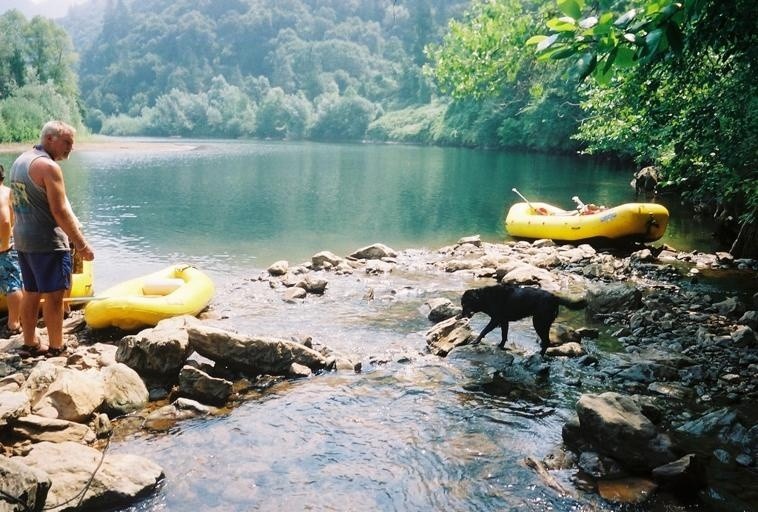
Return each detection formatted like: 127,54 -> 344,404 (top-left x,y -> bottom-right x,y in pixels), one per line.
505,202 -> 669,245
71,260 -> 214,330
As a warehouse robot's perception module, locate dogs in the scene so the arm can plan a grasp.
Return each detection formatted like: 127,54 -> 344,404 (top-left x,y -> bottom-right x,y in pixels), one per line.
460,283 -> 588,357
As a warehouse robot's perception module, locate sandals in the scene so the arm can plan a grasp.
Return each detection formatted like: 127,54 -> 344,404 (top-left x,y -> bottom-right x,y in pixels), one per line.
0,324 -> 23,340
45,345 -> 69,359
20,339 -> 47,358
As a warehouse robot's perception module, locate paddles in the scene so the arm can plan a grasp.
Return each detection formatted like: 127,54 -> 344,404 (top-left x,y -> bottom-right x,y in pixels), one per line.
40,297 -> 111,303
512,188 -> 548,215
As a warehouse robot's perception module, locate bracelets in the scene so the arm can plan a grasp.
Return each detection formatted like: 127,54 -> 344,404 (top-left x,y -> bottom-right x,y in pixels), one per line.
76,244 -> 88,252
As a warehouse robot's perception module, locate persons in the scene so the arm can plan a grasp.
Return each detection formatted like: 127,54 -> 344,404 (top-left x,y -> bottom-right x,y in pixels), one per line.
0,164 -> 24,333
7,119 -> 97,360
